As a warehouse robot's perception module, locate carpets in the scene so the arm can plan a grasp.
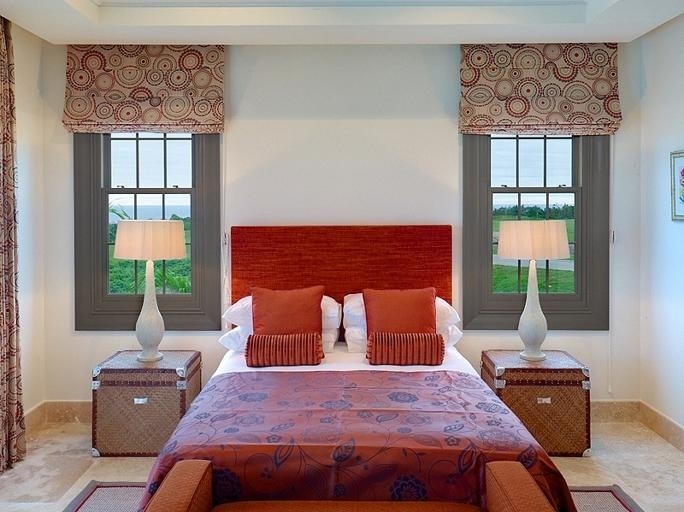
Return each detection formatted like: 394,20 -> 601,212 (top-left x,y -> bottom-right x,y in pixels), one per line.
63,479 -> 148,512
568,484 -> 644,511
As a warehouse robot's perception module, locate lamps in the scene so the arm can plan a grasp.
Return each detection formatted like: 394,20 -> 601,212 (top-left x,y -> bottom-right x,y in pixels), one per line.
113,218 -> 187,362
497,220 -> 570,360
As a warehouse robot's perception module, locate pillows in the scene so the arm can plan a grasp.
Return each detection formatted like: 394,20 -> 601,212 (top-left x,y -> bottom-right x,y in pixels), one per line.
344,327 -> 464,353
221,294 -> 342,331
250,285 -> 326,358
217,327 -> 338,353
366,333 -> 445,366
342,292 -> 461,327
363,288 -> 437,341
245,335 -> 322,367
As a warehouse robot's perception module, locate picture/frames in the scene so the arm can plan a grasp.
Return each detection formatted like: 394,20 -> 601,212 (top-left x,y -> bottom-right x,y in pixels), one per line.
670,150 -> 684,221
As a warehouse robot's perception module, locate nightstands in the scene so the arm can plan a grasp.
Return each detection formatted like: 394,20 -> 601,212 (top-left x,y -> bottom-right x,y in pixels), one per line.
481,350 -> 591,457
91,349 -> 202,457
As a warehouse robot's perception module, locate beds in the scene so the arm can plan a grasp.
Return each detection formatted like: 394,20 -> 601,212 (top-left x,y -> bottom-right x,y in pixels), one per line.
136,223 -> 577,512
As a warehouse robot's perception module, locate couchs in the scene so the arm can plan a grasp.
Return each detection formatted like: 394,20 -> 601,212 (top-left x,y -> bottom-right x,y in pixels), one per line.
144,460 -> 556,512
